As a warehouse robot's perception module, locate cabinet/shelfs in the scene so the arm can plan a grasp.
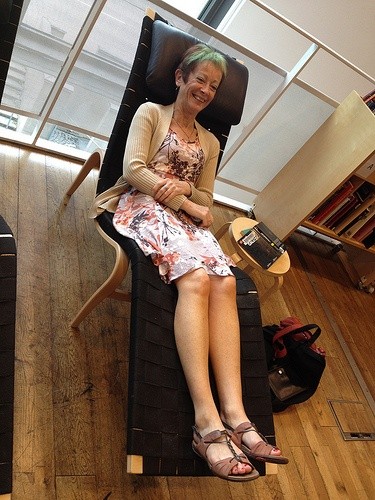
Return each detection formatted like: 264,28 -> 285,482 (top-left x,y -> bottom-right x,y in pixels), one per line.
250,91 -> 375,293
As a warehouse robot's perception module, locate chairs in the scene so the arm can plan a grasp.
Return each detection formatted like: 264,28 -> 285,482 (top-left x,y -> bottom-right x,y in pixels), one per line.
0,0 -> 27,497
65,9 -> 279,478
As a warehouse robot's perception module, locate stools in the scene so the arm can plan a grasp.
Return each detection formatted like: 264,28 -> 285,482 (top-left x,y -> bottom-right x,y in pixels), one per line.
215,217 -> 290,305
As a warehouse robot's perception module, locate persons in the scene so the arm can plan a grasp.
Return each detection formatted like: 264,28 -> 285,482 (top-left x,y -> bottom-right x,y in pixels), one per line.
89,45 -> 289,482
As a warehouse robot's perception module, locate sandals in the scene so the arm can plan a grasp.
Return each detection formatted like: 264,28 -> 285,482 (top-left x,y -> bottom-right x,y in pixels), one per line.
193,426 -> 259,482
221,419 -> 288,465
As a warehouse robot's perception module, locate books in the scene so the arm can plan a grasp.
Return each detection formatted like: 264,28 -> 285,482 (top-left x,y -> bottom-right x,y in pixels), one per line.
309,176 -> 375,249
239,221 -> 287,270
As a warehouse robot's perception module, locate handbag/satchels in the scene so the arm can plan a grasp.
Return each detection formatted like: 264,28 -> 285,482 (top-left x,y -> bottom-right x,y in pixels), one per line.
263,318 -> 325,412
